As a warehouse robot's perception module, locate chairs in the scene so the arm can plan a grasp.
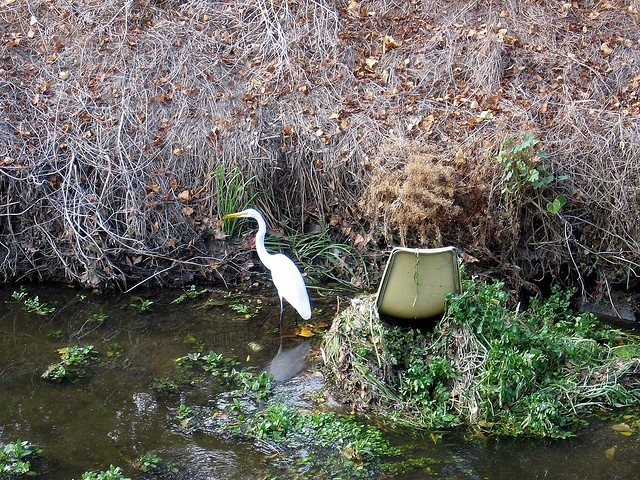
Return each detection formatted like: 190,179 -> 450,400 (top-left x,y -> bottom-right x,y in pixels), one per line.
374,246 -> 463,326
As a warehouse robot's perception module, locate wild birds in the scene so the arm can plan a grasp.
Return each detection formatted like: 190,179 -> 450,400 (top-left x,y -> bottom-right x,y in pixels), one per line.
221,208 -> 312,325
